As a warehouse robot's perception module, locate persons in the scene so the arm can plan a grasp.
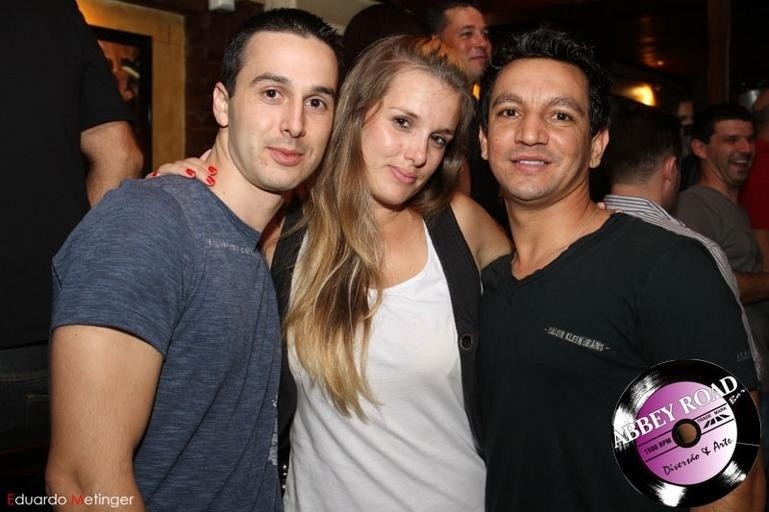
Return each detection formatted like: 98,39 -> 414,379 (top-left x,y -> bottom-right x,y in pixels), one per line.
45,8 -> 346,512
469,31 -> 769,512
341,5 -> 423,65
593,86 -> 769,465
435,1 -> 514,240
143,36 -> 624,511
108,56 -> 140,100
1,1 -> 146,512
